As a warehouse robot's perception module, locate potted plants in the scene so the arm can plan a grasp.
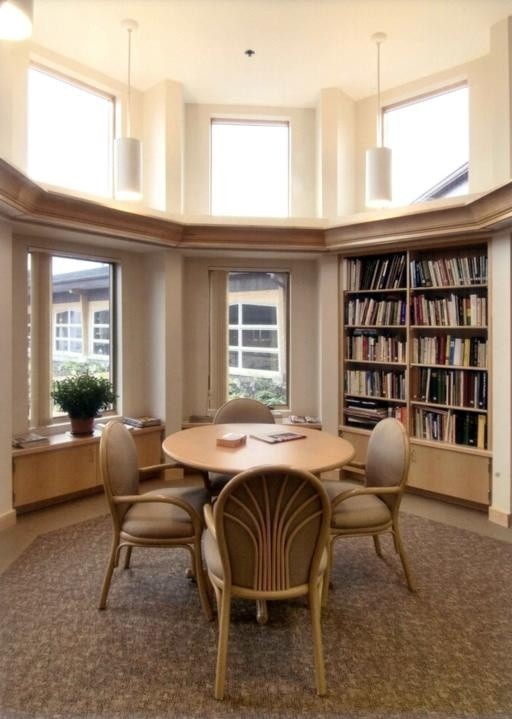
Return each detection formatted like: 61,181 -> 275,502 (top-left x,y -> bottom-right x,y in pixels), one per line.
51,373 -> 120,437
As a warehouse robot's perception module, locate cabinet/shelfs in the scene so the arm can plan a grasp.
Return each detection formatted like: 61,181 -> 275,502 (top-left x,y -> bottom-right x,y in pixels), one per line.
12,432 -> 162,516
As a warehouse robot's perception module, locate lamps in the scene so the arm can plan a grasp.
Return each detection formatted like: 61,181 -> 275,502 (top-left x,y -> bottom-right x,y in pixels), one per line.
364,31 -> 391,202
115,20 -> 141,195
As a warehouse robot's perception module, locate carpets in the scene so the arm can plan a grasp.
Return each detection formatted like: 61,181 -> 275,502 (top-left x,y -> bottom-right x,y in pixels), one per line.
1,514 -> 512,718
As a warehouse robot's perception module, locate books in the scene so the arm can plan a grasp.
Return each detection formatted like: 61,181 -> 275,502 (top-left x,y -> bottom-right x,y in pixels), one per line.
13,432 -> 50,448
340,246 -> 487,454
250,430 -> 305,446
124,415 -> 161,429
187,414 -> 213,423
